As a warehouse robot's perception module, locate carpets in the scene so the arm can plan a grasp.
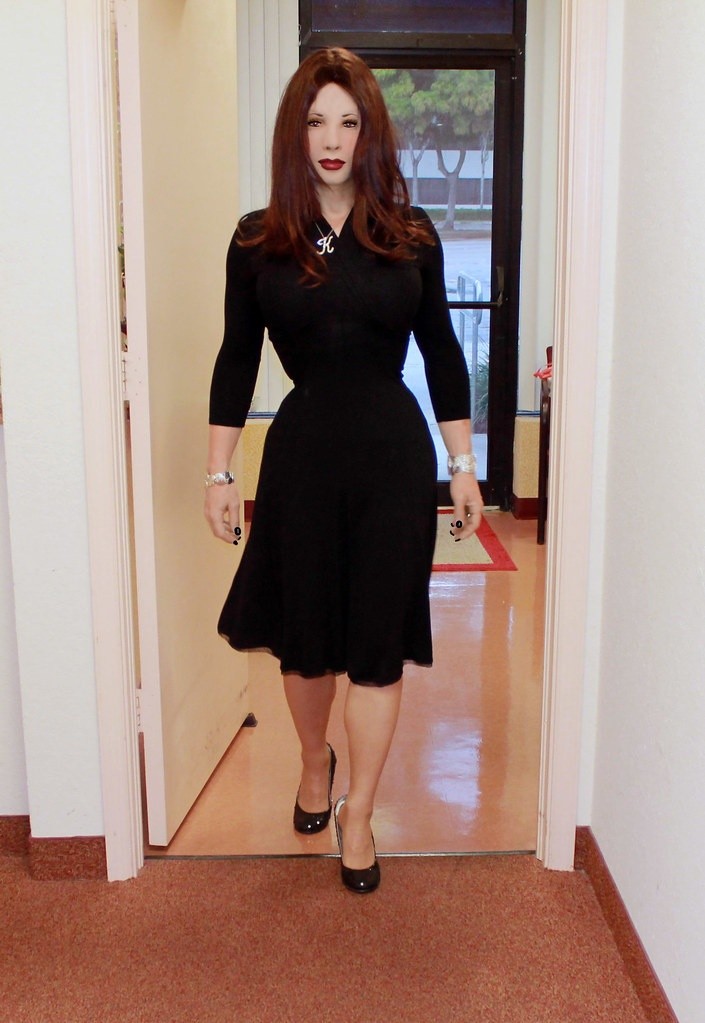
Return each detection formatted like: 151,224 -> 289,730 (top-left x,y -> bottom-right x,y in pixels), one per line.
243,500 -> 519,571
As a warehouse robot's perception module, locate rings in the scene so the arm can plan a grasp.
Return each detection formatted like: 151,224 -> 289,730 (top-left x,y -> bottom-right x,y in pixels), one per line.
467,513 -> 475,518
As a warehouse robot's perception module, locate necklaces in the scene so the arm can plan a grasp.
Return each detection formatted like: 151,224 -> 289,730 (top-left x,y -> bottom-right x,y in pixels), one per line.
313,197 -> 356,255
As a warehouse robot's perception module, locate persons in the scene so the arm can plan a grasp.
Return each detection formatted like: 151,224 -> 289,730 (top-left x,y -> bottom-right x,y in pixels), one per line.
203,46 -> 483,894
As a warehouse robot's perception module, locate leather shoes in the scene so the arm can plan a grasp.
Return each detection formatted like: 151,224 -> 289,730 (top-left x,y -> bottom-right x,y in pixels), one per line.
291,741 -> 337,832
333,792 -> 382,894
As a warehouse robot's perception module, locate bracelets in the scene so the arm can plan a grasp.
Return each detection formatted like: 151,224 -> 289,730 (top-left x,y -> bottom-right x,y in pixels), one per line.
205,471 -> 234,486
447,454 -> 477,475
